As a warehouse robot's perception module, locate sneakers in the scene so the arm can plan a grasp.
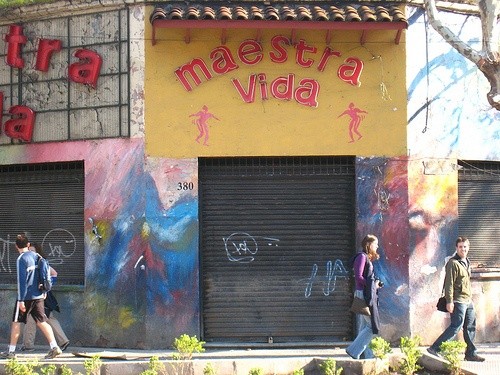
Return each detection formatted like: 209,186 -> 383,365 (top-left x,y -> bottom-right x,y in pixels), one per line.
44,348 -> 61,358
61,341 -> 69,350
1,351 -> 14,359
17,346 -> 34,352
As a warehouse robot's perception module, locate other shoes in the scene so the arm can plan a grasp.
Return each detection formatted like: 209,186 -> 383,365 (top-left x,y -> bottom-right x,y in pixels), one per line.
426,346 -> 486,362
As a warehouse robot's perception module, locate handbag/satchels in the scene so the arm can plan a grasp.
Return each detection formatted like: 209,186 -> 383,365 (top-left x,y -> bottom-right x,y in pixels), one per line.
435,297 -> 449,313
350,296 -> 371,316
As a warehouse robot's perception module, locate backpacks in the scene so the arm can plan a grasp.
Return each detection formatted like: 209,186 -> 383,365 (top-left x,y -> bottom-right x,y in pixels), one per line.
36,255 -> 52,292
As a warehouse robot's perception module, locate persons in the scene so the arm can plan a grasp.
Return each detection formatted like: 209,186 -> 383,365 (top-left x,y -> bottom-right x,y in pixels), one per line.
0,232 -> 62,361
344,235 -> 384,362
425,237 -> 485,364
17,243 -> 72,354
